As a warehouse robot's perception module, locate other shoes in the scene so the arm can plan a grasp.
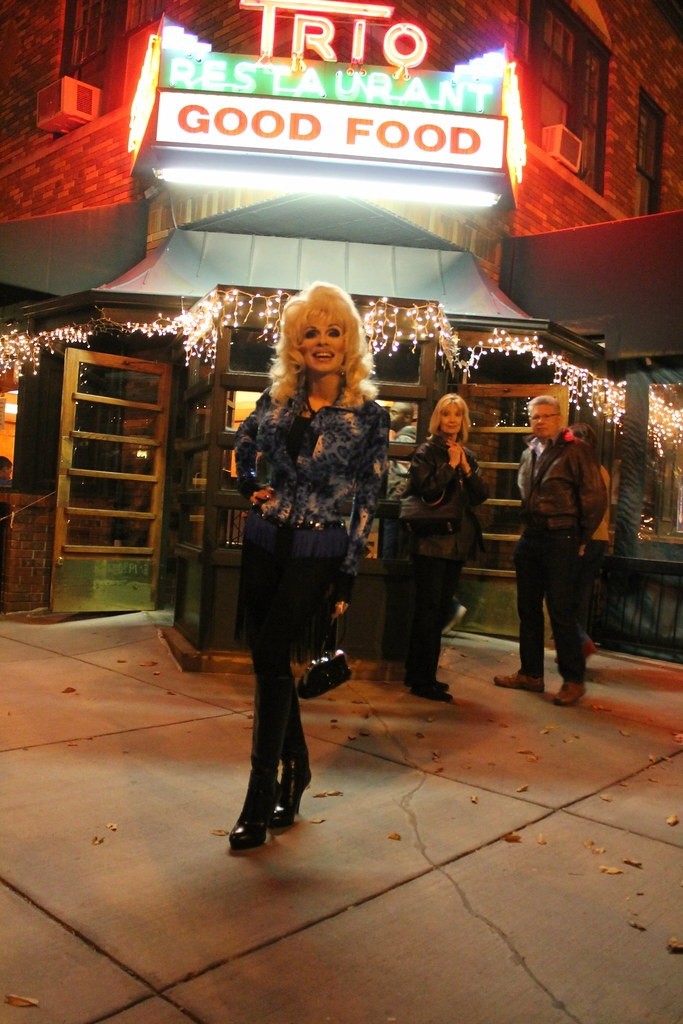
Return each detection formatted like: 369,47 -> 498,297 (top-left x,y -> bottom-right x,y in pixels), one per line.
430,681 -> 449,692
411,683 -> 453,703
493,671 -> 545,693
552,678 -> 587,708
554,639 -> 597,664
440,602 -> 468,640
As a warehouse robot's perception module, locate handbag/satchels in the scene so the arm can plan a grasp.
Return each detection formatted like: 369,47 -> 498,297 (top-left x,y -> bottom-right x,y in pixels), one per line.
575,536 -> 608,583
295,602 -> 352,700
397,463 -> 464,538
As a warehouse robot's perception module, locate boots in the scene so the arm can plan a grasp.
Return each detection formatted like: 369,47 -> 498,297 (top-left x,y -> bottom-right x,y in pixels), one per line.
228,678 -> 285,851
267,676 -> 312,829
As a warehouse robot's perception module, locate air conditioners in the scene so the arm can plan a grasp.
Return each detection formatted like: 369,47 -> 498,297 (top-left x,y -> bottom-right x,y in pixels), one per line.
37,75 -> 101,134
542,125 -> 584,172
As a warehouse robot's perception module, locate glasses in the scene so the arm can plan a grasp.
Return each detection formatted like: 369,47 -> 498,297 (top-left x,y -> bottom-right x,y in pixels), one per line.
528,413 -> 560,421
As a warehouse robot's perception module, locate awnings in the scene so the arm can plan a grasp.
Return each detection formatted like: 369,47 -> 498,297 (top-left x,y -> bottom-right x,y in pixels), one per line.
0,197 -> 148,296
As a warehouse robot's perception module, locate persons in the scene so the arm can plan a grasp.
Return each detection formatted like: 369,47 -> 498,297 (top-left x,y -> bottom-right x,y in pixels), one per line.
406,393 -> 491,703
0,456 -> 13,480
554,424 -> 612,662
230,283 -> 389,849
384,413 -> 417,496
494,396 -> 609,705
